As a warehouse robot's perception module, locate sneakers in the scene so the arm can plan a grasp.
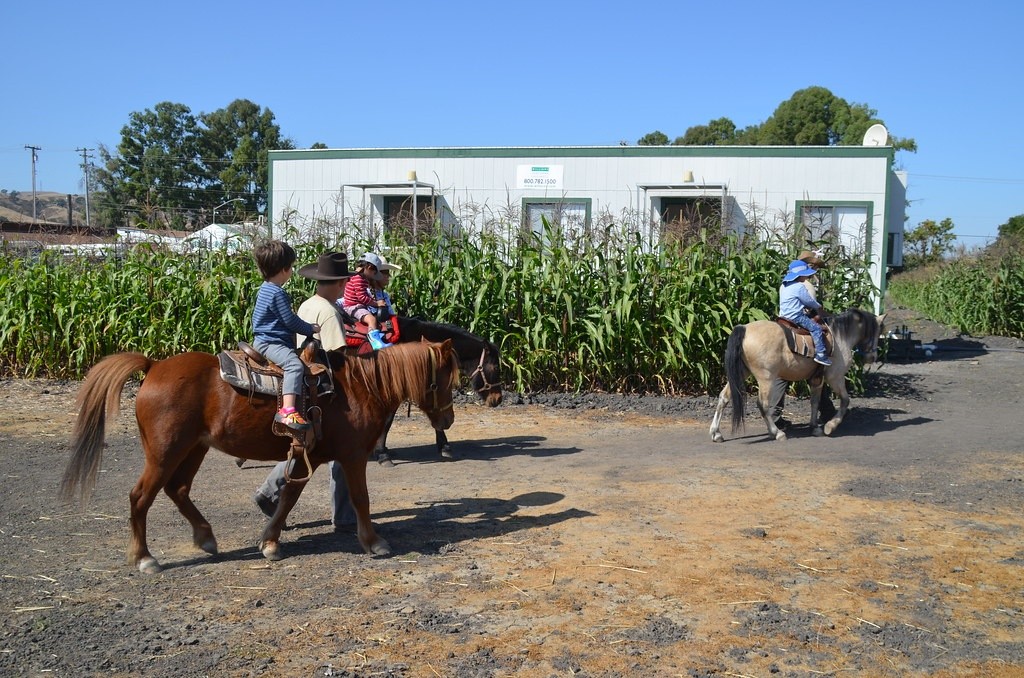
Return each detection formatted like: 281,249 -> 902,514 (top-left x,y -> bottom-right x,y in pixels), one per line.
274,407 -> 309,431
814,354 -> 832,366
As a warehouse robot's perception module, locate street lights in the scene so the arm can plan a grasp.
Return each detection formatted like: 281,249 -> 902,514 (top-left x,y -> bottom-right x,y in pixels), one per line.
212,198 -> 244,224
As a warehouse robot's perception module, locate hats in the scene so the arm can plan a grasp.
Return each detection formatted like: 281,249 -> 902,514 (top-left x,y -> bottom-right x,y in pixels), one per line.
797,250 -> 826,268
783,260 -> 817,281
301,251 -> 357,279
378,257 -> 402,271
360,252 -> 382,273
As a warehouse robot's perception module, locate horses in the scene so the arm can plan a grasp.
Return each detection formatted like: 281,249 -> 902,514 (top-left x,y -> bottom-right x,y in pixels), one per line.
374,317 -> 502,468
54,335 -> 455,574
708,310 -> 888,442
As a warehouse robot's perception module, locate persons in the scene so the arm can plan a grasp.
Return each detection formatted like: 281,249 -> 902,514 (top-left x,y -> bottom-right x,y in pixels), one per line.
774,250 -> 849,430
252,241 -> 320,430
256,253 -> 401,532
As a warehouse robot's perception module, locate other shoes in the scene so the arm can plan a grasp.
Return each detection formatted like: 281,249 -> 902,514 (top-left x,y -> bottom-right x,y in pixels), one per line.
775,419 -> 791,430
256,494 -> 287,530
820,410 -> 837,422
337,521 -> 379,534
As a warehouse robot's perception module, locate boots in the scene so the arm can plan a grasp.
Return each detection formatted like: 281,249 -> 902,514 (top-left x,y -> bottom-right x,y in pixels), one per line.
368,329 -> 392,351
